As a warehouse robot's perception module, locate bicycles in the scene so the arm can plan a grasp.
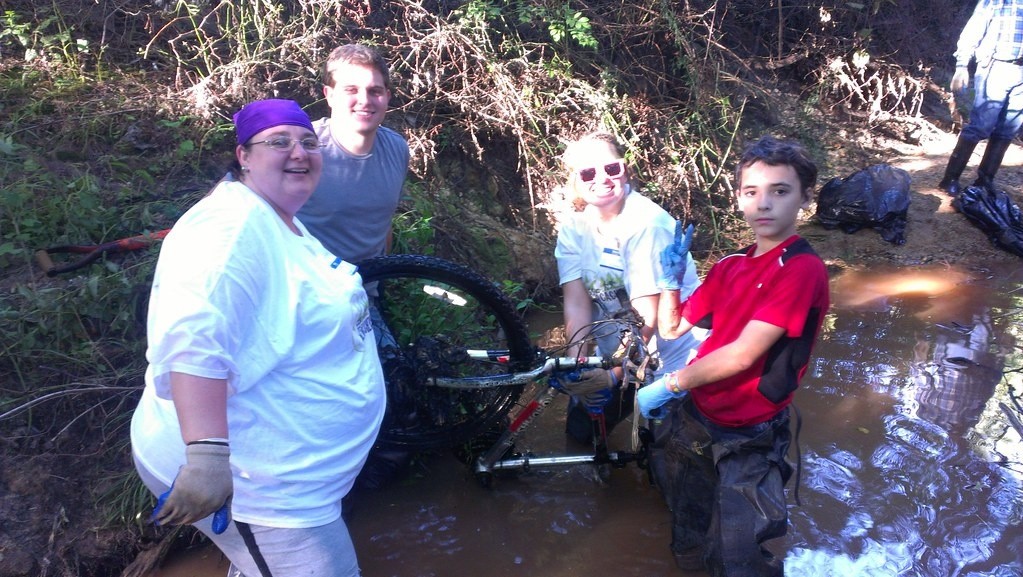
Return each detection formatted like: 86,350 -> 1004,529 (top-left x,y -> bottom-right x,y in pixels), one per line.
346,254 -> 658,492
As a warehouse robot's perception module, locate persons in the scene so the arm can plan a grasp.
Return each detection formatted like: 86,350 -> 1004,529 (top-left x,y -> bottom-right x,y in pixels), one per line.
555,133 -> 712,443
131,99 -> 385,577
635,137 -> 830,576
939,0 -> 1023,195
203,44 -> 410,407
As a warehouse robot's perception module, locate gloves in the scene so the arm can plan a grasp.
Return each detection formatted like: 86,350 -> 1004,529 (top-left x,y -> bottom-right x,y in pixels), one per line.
659,219 -> 694,290
949,67 -> 969,93
151,442 -> 235,534
578,390 -> 613,413
558,368 -> 616,396
636,372 -> 686,419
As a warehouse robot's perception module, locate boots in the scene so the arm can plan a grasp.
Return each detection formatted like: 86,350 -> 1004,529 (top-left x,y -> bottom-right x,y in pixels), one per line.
975,137 -> 1013,195
940,134 -> 978,197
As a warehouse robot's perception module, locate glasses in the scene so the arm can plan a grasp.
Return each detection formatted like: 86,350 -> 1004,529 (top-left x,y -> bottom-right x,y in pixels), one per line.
572,158 -> 627,183
243,137 -> 327,153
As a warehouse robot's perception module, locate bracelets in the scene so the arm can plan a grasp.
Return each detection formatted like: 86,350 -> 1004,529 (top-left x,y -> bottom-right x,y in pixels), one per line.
670,371 -> 683,393
609,367 -> 618,385
